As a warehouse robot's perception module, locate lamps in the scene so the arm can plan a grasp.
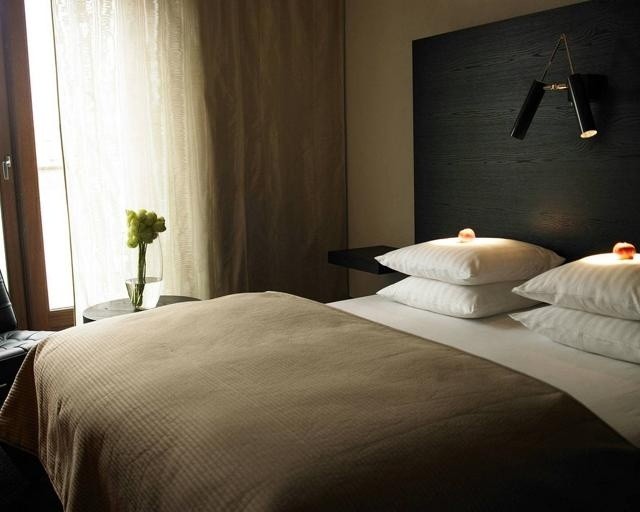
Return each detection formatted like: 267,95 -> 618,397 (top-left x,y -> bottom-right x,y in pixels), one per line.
510,30 -> 597,143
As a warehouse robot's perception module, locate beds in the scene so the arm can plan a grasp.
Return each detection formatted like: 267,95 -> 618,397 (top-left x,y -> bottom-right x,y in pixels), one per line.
34,252 -> 639,512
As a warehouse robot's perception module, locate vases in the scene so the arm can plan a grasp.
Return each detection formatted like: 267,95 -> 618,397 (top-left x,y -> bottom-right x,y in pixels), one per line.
122,231 -> 163,310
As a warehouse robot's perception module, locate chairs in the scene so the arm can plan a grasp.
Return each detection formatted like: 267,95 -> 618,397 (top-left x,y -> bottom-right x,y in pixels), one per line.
0,272 -> 57,382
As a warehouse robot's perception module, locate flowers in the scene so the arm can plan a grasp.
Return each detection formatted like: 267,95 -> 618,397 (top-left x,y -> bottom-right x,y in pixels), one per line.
124,208 -> 167,307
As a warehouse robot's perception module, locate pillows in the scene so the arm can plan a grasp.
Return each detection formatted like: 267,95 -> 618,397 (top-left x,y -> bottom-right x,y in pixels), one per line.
371,231 -> 639,363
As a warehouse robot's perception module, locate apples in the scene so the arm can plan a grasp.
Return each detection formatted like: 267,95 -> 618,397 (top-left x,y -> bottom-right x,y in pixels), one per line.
459,229 -> 476,242
613,243 -> 636,259
